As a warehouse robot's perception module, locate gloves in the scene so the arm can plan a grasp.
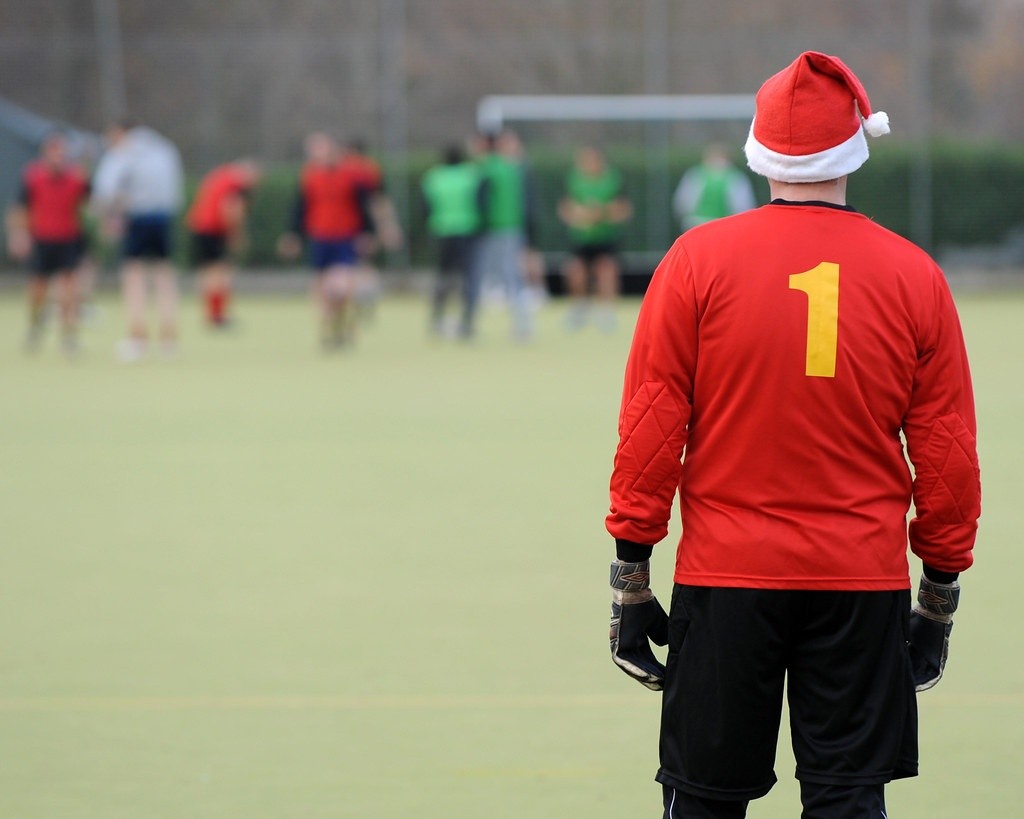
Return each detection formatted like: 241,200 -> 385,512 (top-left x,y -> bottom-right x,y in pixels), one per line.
610,560 -> 670,692
909,573 -> 960,692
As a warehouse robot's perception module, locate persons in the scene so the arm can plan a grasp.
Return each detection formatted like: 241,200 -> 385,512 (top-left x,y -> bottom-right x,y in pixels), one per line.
556,143 -> 633,296
91,118 -> 181,333
672,143 -> 756,232
606,51 -> 981,819
4,134 -> 92,326
182,159 -> 263,323
422,126 -> 540,339
288,129 -> 403,322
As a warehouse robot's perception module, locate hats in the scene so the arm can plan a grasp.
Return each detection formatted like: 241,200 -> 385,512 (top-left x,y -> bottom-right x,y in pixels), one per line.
743,51 -> 891,183
